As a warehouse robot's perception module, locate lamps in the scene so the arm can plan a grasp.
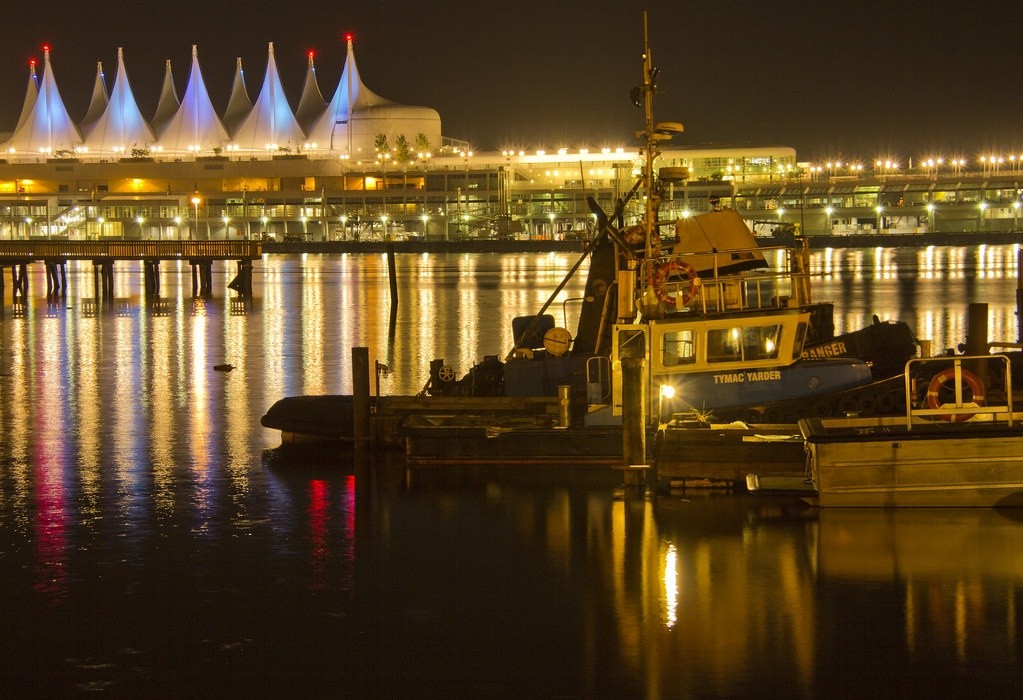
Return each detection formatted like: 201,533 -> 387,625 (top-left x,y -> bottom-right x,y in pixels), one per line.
659,384 -> 675,426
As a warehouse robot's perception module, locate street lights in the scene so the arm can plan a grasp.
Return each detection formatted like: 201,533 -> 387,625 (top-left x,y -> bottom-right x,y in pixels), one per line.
13,141 -> 1021,247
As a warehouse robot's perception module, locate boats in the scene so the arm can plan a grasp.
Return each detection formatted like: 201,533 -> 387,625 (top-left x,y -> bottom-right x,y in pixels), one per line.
254,10 -> 1022,511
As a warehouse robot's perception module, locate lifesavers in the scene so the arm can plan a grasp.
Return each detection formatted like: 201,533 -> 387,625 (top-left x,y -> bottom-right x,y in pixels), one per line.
927,368 -> 985,423
653,260 -> 698,306
